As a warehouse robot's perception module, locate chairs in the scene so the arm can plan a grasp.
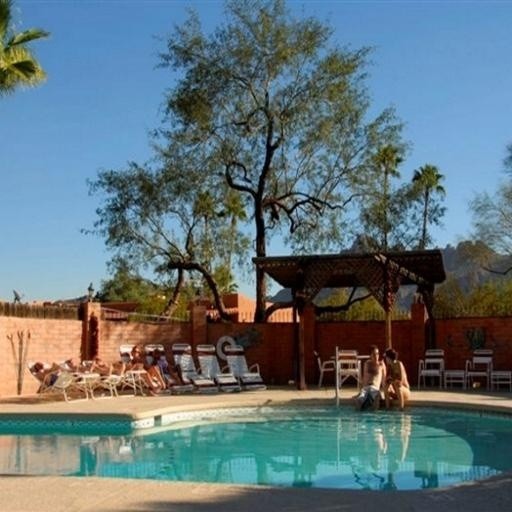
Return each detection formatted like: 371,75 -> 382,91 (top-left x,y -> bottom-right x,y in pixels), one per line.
27,345 -> 266,404
314,346 -> 512,388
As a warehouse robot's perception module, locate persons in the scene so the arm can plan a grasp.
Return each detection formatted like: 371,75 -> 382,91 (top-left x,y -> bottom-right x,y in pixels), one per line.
352,345 -> 387,410
89,435 -> 145,477
388,413 -> 412,472
367,424 -> 388,471
34,345 -> 169,397
383,348 -> 410,411
